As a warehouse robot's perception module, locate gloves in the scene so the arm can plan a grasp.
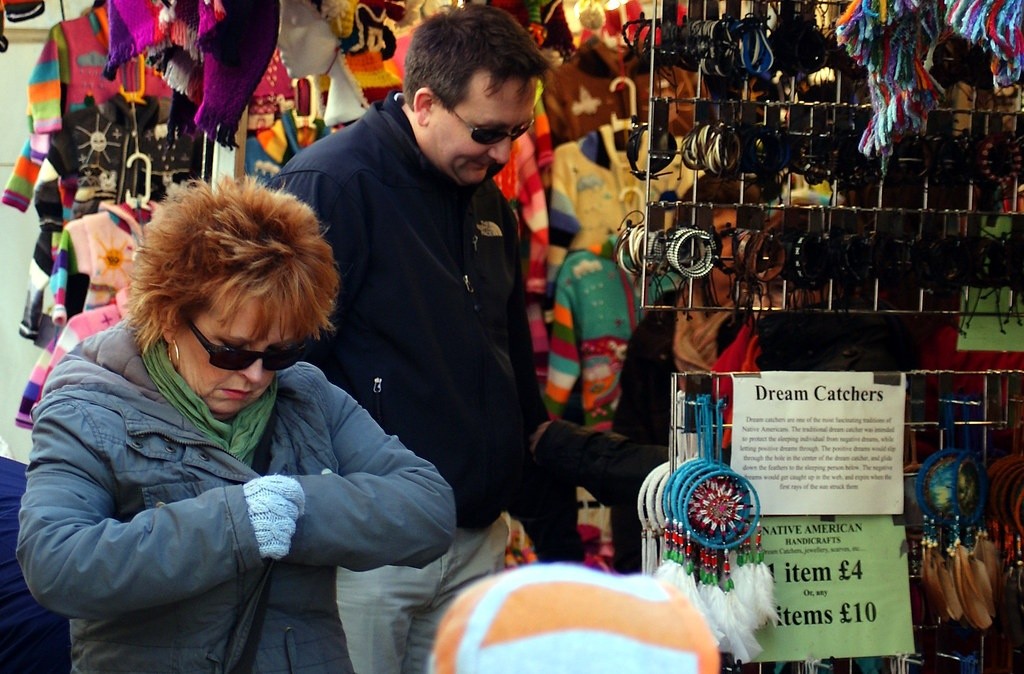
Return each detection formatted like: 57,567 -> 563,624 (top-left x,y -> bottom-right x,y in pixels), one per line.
242,475 -> 305,559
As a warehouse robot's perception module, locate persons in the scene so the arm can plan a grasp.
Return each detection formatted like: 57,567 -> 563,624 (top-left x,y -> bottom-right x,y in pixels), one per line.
530,197 -> 919,510
268,4 -> 586,673
14,176 -> 457,674
611,174 -> 763,574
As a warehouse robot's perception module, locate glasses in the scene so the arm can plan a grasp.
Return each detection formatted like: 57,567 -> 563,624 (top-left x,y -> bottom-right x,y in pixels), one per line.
413,85 -> 534,145
186,318 -> 306,371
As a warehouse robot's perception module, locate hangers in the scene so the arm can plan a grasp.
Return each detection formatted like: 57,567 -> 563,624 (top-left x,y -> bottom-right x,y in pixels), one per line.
120,53 -> 148,105
126,153 -> 152,211
291,75 -> 318,129
619,186 -> 644,222
610,76 -> 637,133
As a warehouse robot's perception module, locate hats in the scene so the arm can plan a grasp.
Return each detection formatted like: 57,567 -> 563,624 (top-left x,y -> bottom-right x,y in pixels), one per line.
429,562 -> 722,674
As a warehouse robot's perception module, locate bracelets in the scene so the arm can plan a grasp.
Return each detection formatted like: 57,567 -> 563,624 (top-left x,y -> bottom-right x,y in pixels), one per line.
615,210 -> 716,283
